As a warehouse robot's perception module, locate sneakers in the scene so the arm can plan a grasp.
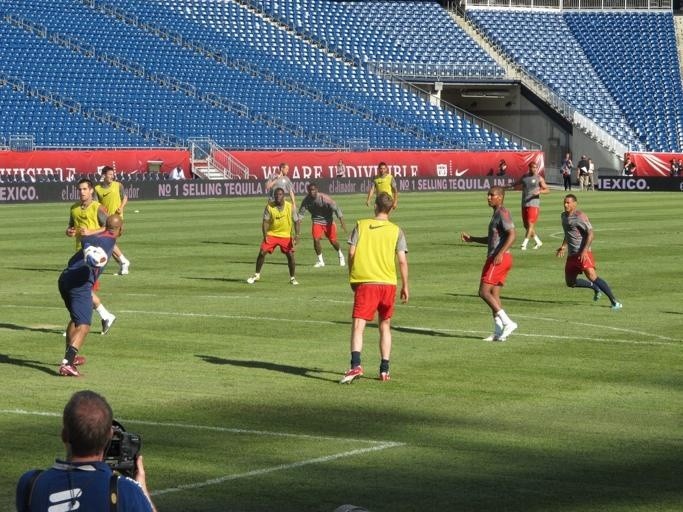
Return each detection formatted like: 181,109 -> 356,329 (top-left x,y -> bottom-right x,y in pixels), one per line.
73,357 -> 85,365
339,364 -> 364,385
247,273 -> 260,284
485,332 -> 507,341
290,277 -> 299,285
121,260 -> 130,275
594,288 -> 602,301
533,241 -> 542,249
339,256 -> 346,266
60,365 -> 81,376
499,321 -> 518,342
521,244 -> 526,250
113,268 -> 129,275
380,372 -> 390,381
314,260 -> 324,267
101,315 -> 116,335
611,303 -> 622,310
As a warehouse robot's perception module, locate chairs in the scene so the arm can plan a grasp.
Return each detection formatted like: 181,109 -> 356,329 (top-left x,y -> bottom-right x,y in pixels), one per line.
74,172 -> 102,183
115,171 -> 170,181
1,174 -> 61,183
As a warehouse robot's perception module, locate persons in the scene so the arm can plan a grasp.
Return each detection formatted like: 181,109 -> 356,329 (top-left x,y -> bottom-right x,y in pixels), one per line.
336,158 -> 347,177
297,183 -> 349,269
55,214 -> 125,377
266,163 -> 297,209
501,160 -> 550,251
246,188 -> 299,287
336,191 -> 410,386
14,388 -> 159,512
93,165 -> 130,275
168,164 -> 187,181
65,177 -> 117,336
669,158 -> 683,177
559,153 -> 637,192
460,185 -> 518,343
553,193 -> 624,310
365,162 -> 398,215
496,159 -> 508,176
